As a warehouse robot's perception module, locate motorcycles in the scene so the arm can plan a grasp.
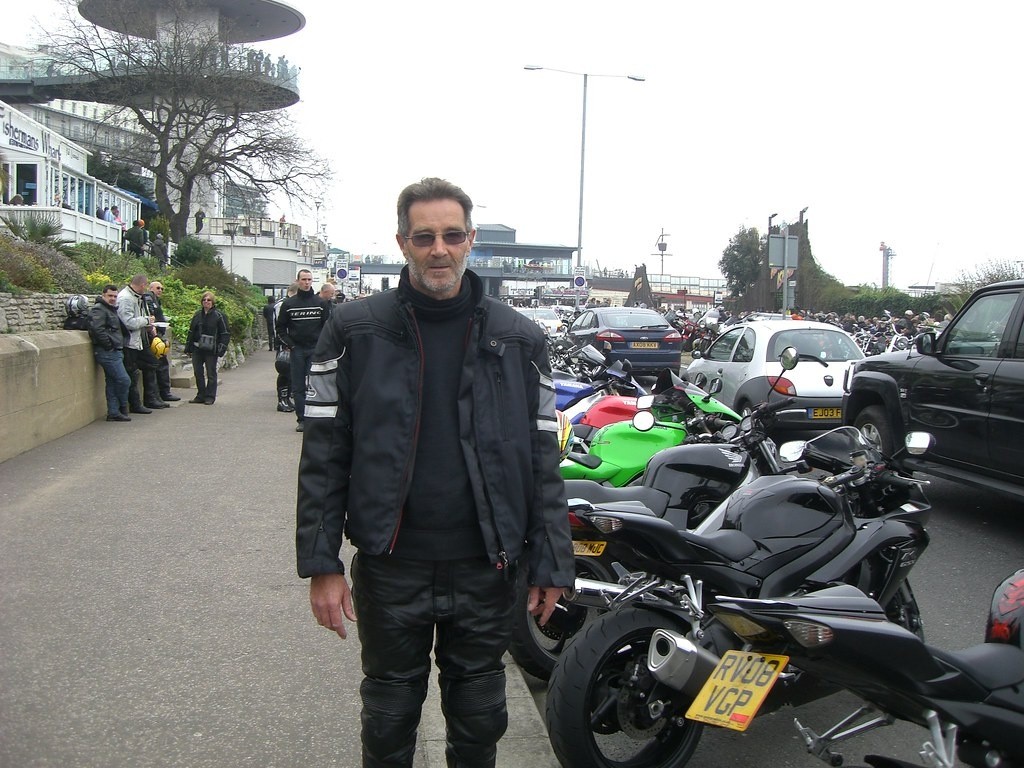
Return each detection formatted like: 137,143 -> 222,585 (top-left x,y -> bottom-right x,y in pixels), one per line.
502,317 -> 937,768
704,563 -> 1023,768
847,310 -> 943,356
665,308 -> 740,353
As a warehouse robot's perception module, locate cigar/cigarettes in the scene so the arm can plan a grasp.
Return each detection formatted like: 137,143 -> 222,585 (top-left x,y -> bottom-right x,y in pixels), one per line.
543,598 -> 568,612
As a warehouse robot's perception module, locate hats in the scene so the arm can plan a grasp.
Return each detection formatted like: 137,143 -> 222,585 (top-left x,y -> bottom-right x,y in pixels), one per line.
157,234 -> 163,239
905,310 -> 913,315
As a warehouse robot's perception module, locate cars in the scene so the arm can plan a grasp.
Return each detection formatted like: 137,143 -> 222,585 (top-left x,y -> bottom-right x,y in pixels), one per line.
682,319 -> 872,442
510,306 -> 561,334
559,307 -> 683,378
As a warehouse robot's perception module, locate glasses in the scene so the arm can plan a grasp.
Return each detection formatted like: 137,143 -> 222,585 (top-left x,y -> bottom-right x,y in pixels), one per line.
404,231 -> 470,247
154,286 -> 163,291
202,299 -> 212,301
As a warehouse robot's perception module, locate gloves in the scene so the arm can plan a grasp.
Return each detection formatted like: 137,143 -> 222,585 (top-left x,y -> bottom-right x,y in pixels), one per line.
218,343 -> 226,357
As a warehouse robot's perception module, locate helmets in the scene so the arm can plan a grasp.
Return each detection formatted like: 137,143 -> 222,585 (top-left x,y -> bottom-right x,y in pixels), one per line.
66,294 -> 89,316
274,350 -> 289,372
150,337 -> 170,358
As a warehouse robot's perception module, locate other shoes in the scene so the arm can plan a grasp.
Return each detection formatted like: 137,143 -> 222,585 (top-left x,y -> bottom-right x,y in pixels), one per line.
163,394 -> 180,401
130,405 -> 152,414
119,405 -> 129,416
107,413 -> 131,421
144,399 -> 163,409
296,421 -> 305,432
158,397 -> 171,409
189,397 -> 204,403
205,399 -> 213,404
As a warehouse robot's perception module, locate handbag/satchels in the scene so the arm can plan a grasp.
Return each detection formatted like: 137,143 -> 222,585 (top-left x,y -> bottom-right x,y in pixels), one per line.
199,334 -> 214,351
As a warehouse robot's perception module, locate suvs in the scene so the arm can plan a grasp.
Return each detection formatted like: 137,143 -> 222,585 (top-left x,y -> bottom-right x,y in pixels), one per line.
841,278 -> 1024,508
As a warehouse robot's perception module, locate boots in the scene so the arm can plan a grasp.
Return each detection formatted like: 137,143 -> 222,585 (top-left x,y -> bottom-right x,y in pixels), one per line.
277,386 -> 296,411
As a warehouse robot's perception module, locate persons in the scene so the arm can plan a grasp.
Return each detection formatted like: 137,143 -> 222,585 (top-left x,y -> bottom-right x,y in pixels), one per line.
297,177 -> 577,768
53,192 -> 73,209
280,214 -> 286,237
632,299 -> 775,329
276,268 -> 334,432
272,282 -> 298,413
8,193 -> 24,205
151,233 -> 168,271
96,204 -> 125,230
124,219 -> 148,259
194,207 -> 206,233
115,273 -> 181,414
586,297 -> 612,308
184,291 -> 231,405
779,306 -> 954,358
314,283 -> 348,309
263,295 -> 278,351
86,284 -> 133,421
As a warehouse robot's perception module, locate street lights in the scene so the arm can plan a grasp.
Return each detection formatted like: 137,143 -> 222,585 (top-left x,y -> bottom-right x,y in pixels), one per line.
320,223 -> 332,249
223,222 -> 241,283
519,64 -> 646,267
315,201 -> 322,239
